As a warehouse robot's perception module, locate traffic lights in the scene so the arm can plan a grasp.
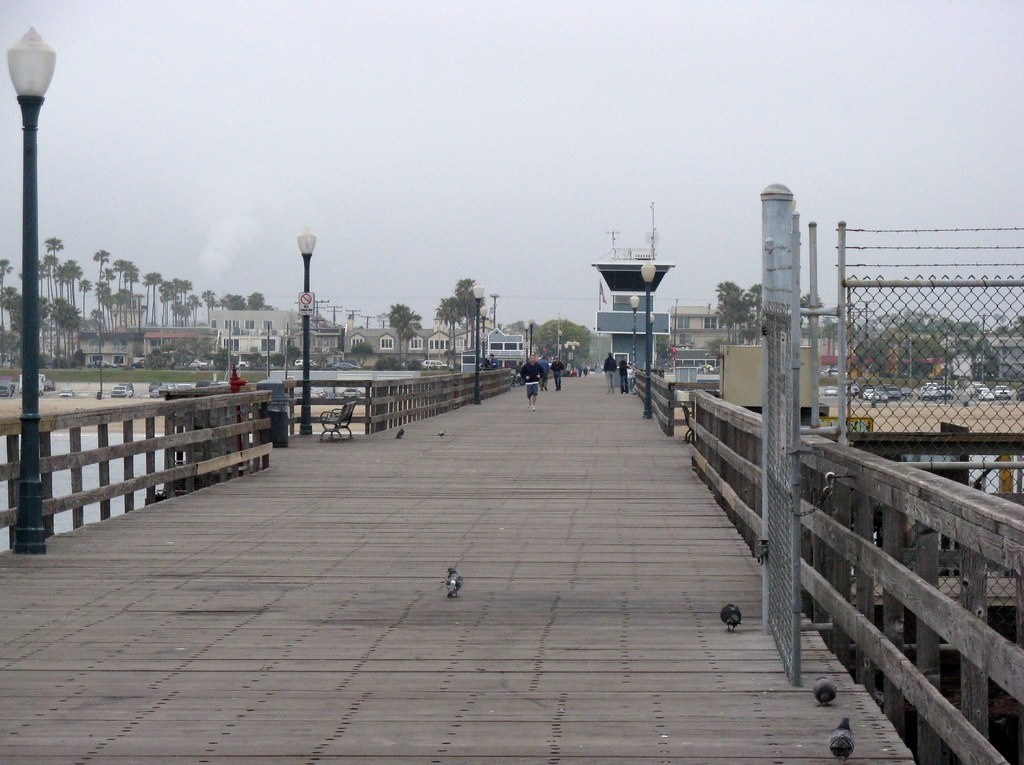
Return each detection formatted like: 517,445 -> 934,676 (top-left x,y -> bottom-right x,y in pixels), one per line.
672,357 -> 675,360
672,349 -> 676,353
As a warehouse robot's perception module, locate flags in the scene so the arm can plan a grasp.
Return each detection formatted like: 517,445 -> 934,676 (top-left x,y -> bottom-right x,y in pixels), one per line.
488,354 -> 499,371
599,279 -> 609,305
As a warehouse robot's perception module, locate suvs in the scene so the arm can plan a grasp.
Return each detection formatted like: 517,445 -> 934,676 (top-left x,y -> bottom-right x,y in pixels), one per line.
420,359 -> 449,370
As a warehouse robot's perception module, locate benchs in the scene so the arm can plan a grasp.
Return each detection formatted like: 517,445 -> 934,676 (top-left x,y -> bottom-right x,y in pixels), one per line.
681,403 -> 695,444
317,401 -> 356,443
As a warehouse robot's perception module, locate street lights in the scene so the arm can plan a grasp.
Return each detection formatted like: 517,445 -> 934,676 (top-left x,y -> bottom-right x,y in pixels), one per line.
480,305 -> 488,362
528,318 -> 535,360
5,26 -> 58,556
557,330 -> 563,362
649,312 -> 655,365
524,321 -> 529,366
295,225 -> 319,436
473,283 -> 484,405
571,345 -> 576,370
640,260 -> 656,420
630,294 -> 639,368
564,343 -> 568,364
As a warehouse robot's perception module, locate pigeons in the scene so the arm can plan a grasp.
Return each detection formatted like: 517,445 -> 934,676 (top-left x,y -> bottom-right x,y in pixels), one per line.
829,716 -> 855,765
438,430 -> 446,438
812,676 -> 836,706
445,568 -> 463,597
719,604 -> 743,631
396,428 -> 404,440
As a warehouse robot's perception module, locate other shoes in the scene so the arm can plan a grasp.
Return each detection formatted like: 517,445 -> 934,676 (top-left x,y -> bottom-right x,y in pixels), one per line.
527,404 -> 535,411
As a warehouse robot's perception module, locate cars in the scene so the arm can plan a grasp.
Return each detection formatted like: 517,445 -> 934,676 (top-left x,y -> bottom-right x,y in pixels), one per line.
91,358 -> 362,372
820,365 -> 1024,404
0,374 -> 362,399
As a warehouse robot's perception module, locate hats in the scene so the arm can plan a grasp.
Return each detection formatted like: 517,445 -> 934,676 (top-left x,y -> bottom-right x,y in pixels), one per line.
489,354 -> 494,356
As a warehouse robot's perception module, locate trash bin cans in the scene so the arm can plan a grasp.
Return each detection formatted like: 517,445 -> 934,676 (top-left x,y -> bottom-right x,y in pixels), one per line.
256,379 -> 293,448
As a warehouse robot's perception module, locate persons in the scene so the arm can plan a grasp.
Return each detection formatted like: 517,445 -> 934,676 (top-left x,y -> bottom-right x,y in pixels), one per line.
603,351 -> 616,393
551,357 -> 564,391
521,356 -> 542,411
567,362 -> 600,377
537,358 -> 548,392
620,356 -> 628,394
